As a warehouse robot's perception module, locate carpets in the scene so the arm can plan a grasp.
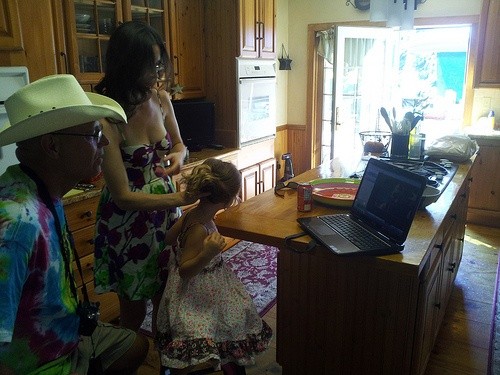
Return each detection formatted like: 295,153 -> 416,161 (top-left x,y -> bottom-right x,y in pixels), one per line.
487,252 -> 500,375
109,240 -> 277,340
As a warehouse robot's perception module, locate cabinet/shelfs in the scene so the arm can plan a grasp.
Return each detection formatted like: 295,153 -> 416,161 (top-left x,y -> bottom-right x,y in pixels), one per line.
0,0 -> 276,289
467,0 -> 500,228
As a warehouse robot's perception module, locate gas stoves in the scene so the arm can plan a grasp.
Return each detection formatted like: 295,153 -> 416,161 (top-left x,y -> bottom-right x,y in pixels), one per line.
350,159 -> 458,201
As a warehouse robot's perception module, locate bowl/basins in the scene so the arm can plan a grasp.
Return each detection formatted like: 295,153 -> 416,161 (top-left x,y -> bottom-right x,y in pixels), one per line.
358,130 -> 392,153
417,185 -> 441,210
75,14 -> 91,24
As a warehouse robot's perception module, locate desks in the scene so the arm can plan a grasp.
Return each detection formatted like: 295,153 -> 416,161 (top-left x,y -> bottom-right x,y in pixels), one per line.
213,147 -> 476,375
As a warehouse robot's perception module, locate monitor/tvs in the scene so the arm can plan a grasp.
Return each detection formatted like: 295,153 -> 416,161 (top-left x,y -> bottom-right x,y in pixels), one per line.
173,102 -> 215,152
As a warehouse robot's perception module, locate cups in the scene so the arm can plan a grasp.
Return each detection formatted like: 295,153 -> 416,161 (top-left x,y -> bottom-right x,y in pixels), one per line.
79,55 -> 98,72
410,133 -> 426,161
390,133 -> 409,159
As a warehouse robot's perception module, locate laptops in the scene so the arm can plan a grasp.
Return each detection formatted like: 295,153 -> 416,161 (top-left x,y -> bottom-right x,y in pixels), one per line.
296,158 -> 427,255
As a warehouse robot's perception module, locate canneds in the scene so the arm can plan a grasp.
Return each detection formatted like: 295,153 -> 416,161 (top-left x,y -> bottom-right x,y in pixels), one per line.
296,182 -> 313,212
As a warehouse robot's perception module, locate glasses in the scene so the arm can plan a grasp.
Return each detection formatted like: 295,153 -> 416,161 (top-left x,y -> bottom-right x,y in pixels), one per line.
58,125 -> 103,142
144,64 -> 164,72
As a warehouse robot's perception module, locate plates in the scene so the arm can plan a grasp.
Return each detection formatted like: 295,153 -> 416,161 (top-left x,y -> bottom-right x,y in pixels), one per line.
307,178 -> 362,206
76,24 -> 92,33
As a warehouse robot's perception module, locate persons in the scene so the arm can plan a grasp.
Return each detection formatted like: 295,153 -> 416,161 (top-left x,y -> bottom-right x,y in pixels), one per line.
93,22 -> 213,335
0,74 -> 149,375
157,158 -> 273,375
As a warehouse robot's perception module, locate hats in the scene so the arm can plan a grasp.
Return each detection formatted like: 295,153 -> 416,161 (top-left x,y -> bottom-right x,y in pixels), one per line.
0,74 -> 127,148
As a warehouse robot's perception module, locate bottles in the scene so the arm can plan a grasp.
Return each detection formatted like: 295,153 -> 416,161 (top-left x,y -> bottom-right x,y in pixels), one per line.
100,17 -> 114,35
488,110 -> 495,129
